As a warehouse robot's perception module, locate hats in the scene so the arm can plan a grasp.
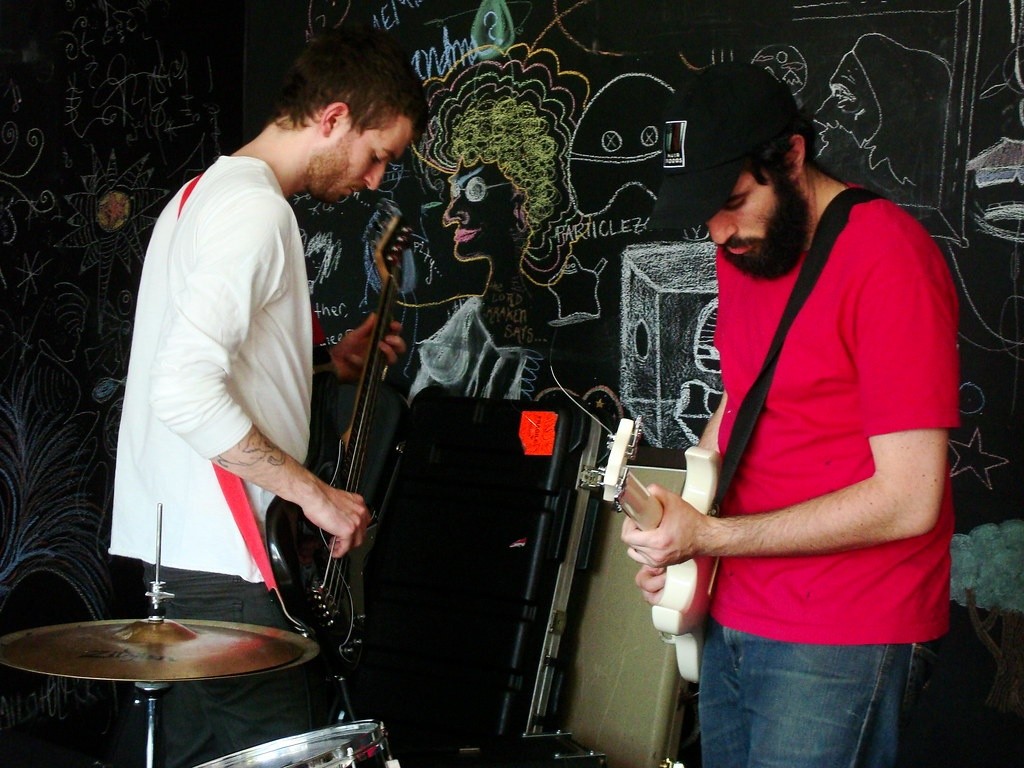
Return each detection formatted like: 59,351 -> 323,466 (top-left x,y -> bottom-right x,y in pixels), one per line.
645,63 -> 799,231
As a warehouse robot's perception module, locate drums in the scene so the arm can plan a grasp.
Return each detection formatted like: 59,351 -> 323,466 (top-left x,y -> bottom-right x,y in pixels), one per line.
191,716 -> 394,768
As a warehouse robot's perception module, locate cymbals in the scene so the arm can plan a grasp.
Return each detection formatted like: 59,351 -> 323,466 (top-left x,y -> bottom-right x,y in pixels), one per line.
0,604 -> 322,685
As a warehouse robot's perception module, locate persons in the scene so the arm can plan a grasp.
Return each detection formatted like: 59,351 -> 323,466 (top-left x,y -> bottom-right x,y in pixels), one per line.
106,27 -> 429,768
621,60 -> 958,768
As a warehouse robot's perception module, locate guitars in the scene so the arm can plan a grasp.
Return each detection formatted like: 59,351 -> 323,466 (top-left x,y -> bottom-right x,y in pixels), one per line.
578,412 -> 721,686
262,206 -> 416,689
